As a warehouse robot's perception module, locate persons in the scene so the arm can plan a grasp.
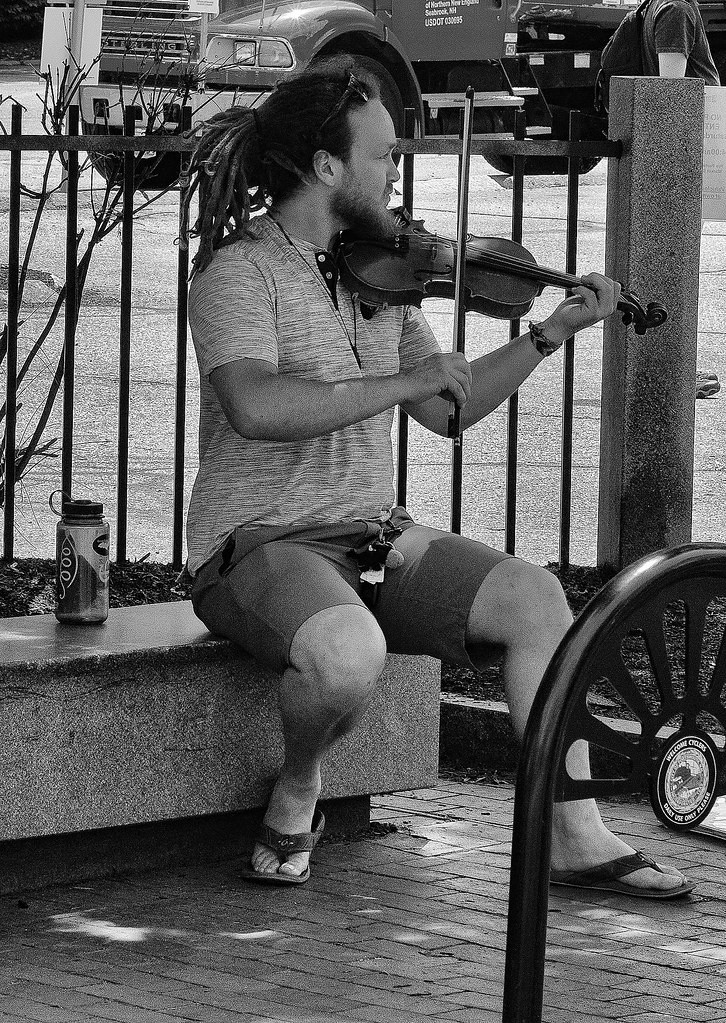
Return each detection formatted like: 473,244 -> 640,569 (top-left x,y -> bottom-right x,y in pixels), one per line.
176,56 -> 696,900
641,0 -> 721,398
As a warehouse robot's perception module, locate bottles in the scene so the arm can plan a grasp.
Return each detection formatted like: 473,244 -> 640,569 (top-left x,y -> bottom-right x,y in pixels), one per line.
49,490 -> 110,624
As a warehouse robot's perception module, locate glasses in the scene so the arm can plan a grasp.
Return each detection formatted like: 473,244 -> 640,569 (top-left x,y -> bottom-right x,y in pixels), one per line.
311,70 -> 368,139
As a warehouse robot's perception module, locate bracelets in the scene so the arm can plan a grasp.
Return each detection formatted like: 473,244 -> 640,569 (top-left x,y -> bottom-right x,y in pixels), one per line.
528,321 -> 563,357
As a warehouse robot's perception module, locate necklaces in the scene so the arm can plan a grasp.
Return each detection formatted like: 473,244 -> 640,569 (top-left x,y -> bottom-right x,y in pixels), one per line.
267,211 -> 362,371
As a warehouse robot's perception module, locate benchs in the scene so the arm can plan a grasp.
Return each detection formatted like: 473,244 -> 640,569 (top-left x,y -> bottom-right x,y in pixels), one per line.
0,601 -> 440,896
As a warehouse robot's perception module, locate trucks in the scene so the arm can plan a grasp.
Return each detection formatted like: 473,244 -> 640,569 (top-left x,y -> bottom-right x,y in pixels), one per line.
41,0 -> 726,191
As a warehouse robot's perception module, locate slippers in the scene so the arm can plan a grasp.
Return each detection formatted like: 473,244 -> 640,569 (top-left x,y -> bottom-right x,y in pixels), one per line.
239,807 -> 325,883
549,847 -> 697,898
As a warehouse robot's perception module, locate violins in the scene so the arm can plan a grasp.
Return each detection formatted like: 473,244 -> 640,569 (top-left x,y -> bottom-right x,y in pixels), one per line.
332,204 -> 672,338
